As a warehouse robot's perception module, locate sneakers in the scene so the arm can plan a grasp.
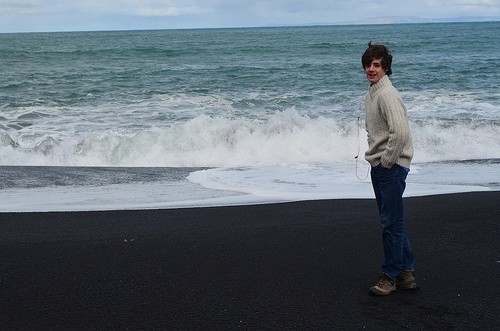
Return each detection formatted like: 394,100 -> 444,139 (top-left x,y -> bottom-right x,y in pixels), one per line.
395,271 -> 416,290
368,272 -> 397,295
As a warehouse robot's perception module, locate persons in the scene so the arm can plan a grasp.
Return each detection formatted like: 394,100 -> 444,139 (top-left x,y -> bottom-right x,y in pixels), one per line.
360,41 -> 417,294
